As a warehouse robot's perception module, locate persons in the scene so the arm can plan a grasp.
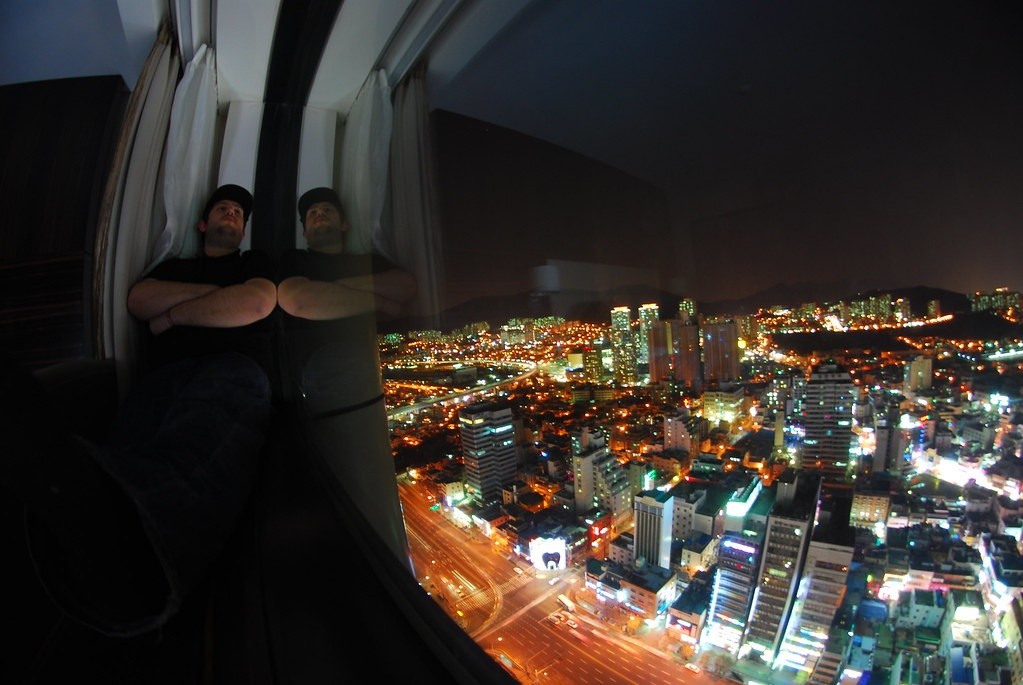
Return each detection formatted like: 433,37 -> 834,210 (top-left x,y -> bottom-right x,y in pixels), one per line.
277,185 -> 419,440
0,183 -> 283,640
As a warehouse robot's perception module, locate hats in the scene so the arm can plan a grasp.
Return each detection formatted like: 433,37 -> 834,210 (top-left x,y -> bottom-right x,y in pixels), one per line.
202,184 -> 254,224
298,187 -> 340,213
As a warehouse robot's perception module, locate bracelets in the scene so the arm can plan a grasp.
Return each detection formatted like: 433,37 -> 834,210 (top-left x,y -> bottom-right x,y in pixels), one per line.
166,309 -> 175,324
